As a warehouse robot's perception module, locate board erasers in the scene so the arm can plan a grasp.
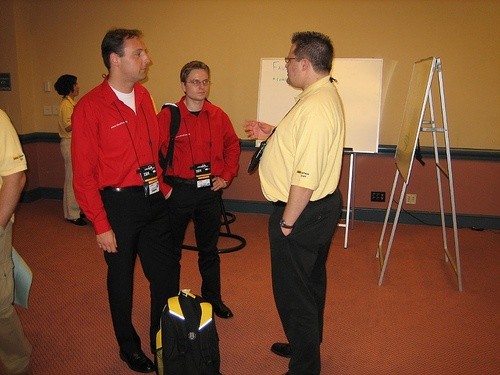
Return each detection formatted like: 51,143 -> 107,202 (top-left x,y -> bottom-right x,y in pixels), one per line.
343,148 -> 353,152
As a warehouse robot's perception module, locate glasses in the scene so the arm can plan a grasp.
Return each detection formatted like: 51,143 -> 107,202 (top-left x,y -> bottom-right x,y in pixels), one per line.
186,79 -> 211,85
285,57 -> 296,63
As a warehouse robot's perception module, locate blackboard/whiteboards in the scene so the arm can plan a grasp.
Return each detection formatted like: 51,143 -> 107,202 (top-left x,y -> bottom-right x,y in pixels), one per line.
393,55 -> 437,184
255,57 -> 383,154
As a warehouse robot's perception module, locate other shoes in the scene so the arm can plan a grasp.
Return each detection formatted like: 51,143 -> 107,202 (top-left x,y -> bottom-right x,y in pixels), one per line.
67,213 -> 87,225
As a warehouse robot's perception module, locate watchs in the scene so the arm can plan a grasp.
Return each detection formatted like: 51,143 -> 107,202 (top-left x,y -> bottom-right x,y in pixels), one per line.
280,220 -> 293,229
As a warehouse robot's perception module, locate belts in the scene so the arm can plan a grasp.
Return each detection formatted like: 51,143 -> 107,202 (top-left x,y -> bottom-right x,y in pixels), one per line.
105,186 -> 143,193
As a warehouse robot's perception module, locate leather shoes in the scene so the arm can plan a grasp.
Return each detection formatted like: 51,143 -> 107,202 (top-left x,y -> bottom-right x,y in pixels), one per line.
119,341 -> 155,373
213,301 -> 233,319
271,342 -> 293,358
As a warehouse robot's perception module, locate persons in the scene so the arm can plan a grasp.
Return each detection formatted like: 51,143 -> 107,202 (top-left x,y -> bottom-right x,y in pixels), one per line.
246,31 -> 345,375
0,109 -> 33,375
54,75 -> 86,226
155,61 -> 241,319
71,29 -> 176,373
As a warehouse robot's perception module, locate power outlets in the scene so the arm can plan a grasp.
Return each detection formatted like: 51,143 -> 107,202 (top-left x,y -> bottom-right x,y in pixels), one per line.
371,192 -> 385,202
406,194 -> 417,204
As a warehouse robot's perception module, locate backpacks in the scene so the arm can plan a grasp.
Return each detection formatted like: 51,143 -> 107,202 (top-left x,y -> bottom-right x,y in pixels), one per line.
153,289 -> 223,375
